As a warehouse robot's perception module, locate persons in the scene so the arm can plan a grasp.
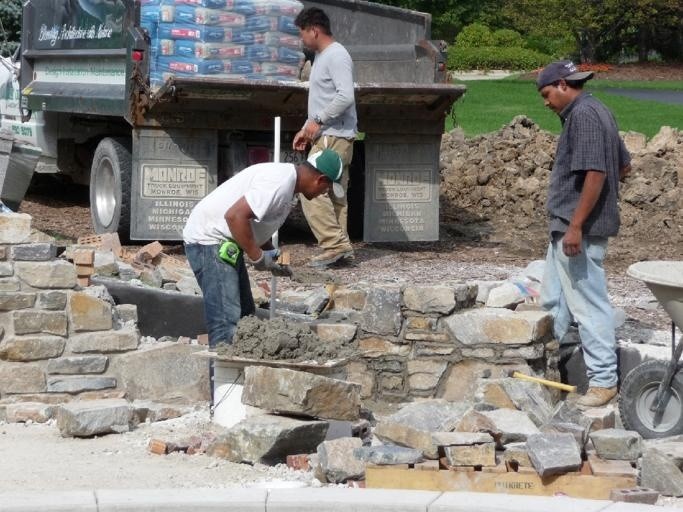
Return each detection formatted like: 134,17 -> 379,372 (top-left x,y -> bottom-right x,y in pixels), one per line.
291,7 -> 361,270
183,150 -> 344,417
537,60 -> 635,408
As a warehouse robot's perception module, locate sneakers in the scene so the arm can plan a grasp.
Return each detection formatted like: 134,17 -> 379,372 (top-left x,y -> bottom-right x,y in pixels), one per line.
306,247 -> 355,267
576,385 -> 617,406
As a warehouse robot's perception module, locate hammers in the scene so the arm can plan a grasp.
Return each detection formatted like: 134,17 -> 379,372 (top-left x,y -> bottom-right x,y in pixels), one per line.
508,369 -> 577,393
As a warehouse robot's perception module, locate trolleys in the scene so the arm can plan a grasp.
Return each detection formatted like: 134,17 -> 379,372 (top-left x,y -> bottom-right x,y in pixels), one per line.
619,259 -> 683,439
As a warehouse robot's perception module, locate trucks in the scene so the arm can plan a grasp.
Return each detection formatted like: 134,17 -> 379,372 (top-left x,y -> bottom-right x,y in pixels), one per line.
0,1 -> 468,242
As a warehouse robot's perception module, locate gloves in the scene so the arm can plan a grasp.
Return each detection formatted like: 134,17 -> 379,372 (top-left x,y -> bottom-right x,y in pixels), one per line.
274,264 -> 295,279
248,248 -> 281,270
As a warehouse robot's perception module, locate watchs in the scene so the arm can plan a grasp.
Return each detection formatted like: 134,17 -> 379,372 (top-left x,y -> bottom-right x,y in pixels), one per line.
314,116 -> 326,125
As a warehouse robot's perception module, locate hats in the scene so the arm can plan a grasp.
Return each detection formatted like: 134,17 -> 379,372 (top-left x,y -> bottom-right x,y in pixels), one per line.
305,148 -> 345,197
535,60 -> 595,92
